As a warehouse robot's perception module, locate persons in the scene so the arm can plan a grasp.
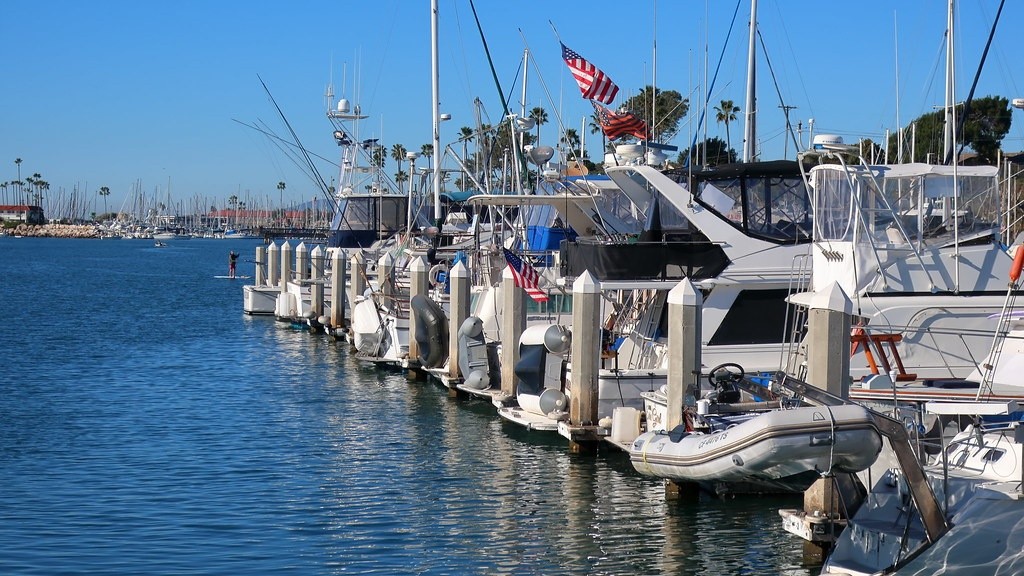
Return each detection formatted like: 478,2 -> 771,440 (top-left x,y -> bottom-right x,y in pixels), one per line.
228,251 -> 239,277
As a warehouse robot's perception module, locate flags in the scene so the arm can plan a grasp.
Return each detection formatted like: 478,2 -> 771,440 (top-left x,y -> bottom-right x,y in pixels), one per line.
560,40 -> 619,105
591,103 -> 655,142
504,247 -> 555,301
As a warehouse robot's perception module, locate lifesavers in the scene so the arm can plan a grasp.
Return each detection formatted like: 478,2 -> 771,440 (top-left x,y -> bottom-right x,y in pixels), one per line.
429,264 -> 449,288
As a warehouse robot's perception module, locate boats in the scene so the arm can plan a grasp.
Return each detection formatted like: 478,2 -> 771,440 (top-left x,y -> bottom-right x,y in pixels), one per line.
630,362 -> 883,489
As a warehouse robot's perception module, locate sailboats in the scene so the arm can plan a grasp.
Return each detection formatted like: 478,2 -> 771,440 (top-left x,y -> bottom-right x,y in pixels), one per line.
44,176 -> 331,239
243,0 -> 1024,576
